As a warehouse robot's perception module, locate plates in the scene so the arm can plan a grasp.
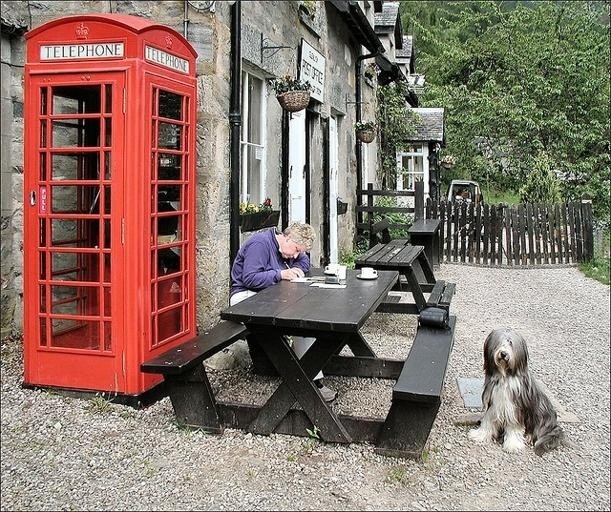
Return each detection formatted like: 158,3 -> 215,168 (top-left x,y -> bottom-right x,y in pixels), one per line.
324,271 -> 338,275
356,274 -> 379,280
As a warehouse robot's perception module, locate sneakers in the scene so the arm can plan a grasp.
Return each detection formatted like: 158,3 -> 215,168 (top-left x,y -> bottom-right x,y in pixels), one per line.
317,383 -> 339,401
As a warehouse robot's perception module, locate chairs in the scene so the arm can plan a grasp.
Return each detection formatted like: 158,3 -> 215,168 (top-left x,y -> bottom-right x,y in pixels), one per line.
370,218 -> 409,248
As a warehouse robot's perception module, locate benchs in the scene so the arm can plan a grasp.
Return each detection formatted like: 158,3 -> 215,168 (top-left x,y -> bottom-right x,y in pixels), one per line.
427,279 -> 458,313
141,323 -> 247,433
373,312 -> 457,456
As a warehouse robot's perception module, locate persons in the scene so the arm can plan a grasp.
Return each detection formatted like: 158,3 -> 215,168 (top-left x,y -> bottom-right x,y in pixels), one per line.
228,221 -> 342,403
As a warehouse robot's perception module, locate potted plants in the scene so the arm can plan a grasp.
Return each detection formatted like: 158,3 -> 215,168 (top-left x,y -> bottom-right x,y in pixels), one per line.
267,76 -> 314,112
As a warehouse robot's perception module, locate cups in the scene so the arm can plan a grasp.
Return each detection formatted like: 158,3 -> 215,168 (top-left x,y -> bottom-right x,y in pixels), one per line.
361,267 -> 378,277
325,263 -> 340,272
335,266 -> 347,280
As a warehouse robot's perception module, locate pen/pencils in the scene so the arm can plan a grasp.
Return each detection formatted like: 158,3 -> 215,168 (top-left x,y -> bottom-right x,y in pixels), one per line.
284,261 -> 293,269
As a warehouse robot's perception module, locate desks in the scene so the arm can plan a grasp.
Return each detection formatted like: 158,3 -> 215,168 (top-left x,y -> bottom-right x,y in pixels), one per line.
407,218 -> 442,272
354,243 -> 436,314
220,267 -> 400,445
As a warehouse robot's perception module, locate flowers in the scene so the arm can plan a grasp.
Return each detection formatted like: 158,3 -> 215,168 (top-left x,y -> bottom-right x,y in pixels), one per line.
240,197 -> 272,215
354,121 -> 375,130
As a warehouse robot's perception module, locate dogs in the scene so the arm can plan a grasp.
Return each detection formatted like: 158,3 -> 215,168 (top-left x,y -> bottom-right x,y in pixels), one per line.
467,327 -> 566,459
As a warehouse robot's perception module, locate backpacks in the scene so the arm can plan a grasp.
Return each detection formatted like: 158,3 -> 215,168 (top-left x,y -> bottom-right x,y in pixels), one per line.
419,305 -> 453,330
